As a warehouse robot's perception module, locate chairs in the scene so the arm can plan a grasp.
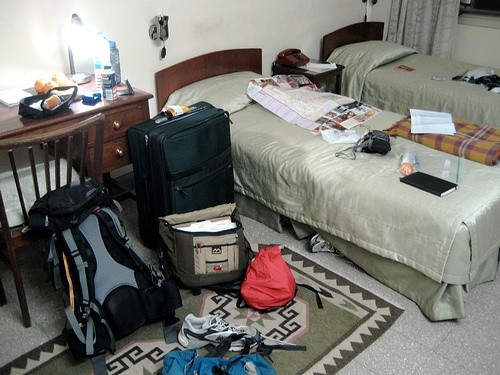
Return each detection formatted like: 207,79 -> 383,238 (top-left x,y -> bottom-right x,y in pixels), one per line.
2,111 -> 104,329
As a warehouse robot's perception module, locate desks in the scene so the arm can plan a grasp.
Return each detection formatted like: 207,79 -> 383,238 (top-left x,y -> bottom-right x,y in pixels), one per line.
0,72 -> 152,307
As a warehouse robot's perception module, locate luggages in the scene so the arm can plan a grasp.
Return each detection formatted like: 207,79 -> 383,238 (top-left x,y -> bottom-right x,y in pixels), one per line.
126,102 -> 236,251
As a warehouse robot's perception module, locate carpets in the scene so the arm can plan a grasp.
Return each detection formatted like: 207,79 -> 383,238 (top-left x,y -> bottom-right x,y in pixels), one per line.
0,242 -> 403,375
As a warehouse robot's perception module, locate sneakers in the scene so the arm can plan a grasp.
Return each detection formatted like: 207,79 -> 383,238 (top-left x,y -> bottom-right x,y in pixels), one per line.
308,233 -> 343,255
179,313 -> 260,353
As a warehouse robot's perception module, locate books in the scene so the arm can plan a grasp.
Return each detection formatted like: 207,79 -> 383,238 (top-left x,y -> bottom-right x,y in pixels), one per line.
400,169 -> 458,199
394,64 -> 415,73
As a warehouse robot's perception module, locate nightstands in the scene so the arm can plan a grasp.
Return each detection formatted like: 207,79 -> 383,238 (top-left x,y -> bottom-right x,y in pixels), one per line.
274,58 -> 344,96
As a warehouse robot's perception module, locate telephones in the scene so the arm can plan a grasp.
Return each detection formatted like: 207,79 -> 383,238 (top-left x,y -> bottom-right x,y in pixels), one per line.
278,48 -> 309,68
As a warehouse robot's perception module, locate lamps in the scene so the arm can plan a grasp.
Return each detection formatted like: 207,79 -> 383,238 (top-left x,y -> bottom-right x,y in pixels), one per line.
65,14 -> 92,86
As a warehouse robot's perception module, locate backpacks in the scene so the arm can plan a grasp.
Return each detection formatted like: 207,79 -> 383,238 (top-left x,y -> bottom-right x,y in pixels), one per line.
26,178 -> 182,375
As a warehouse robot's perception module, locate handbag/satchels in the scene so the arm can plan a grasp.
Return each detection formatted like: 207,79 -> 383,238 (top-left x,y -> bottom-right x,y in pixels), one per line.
160,334 -> 277,375
157,203 -> 251,290
335,130 -> 392,161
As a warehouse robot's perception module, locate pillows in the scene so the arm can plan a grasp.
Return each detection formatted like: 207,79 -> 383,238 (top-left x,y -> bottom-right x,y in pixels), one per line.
326,40 -> 417,70
164,70 -> 268,116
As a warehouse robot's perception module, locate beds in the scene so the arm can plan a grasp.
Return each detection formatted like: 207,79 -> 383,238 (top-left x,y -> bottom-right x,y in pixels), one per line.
154,48 -> 500,323
322,23 -> 500,127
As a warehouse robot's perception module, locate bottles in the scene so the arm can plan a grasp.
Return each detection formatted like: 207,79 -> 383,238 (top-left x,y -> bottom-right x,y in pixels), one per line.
101,65 -> 117,101
94,63 -> 103,90
108,41 -> 122,86
